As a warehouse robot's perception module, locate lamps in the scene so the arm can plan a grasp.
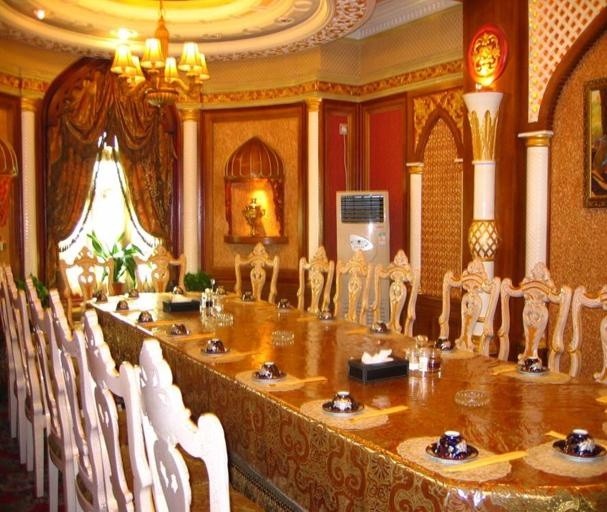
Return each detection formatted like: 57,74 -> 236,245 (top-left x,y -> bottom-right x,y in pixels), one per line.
99,1 -> 212,111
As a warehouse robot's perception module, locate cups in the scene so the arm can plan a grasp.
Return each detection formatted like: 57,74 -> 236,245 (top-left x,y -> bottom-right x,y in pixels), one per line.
94,279 -> 390,336
204,339 -> 226,350
331,391 -> 358,411
562,429 -> 594,455
431,336 -> 450,350
258,362 -> 280,377
437,430 -> 467,458
523,357 -> 543,371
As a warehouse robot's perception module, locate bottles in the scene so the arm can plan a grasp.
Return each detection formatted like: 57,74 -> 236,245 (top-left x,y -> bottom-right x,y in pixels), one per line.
402,344 -> 441,372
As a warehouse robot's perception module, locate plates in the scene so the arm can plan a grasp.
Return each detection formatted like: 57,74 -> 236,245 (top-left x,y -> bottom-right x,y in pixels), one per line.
201,347 -> 228,356
426,441 -> 477,463
551,440 -> 606,463
322,401 -> 365,416
515,364 -> 548,376
251,370 -> 287,382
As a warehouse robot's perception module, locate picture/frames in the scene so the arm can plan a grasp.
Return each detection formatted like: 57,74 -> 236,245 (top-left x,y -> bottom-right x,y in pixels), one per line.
582,75 -> 606,210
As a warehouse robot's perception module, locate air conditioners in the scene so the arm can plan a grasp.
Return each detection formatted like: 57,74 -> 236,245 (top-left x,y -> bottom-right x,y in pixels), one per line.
333,188 -> 391,329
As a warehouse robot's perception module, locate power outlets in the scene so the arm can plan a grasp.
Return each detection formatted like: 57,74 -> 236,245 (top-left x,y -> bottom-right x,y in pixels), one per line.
337,122 -> 348,136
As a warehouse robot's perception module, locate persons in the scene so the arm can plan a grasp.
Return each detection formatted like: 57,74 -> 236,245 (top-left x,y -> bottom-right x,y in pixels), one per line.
245,197 -> 265,233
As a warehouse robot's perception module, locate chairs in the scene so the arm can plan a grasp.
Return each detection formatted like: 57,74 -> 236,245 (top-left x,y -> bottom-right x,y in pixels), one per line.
229,241 -> 607,386
56,245 -> 119,331
0,259 -> 238,511
124,244 -> 188,293
